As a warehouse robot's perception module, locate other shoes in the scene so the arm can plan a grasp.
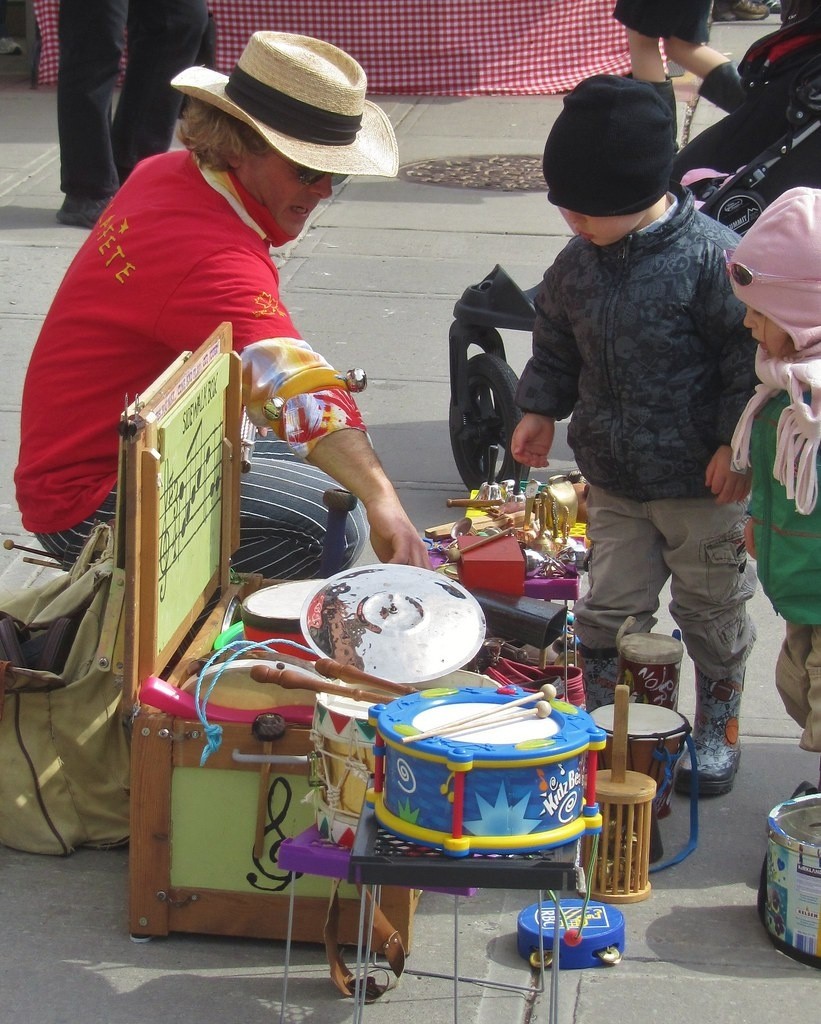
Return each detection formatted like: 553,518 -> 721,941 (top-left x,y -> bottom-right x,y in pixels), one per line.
712,0 -> 770,22
56,182 -> 122,230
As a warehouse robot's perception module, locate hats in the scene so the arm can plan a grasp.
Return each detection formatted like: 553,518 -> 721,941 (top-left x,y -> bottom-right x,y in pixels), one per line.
730,187 -> 821,515
542,73 -> 680,217
170,31 -> 400,178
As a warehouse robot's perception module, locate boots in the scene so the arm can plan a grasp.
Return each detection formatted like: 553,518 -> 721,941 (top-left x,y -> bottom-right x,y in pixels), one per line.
697,60 -> 750,115
673,663 -> 747,796
625,70 -> 676,136
576,642 -> 618,713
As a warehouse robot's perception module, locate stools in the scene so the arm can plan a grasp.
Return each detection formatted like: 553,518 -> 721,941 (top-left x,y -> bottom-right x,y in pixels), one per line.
349,795 -> 579,1024
278,822 -> 489,1024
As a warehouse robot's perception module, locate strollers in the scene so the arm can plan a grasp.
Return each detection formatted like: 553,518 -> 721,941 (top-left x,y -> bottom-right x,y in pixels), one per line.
450,0 -> 821,495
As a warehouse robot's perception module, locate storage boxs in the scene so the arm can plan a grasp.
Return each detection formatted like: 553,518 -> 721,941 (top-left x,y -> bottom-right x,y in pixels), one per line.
117,321 -> 424,961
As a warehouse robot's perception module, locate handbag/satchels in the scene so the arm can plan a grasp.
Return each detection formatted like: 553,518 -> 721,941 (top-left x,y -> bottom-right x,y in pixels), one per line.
0,520 -> 134,855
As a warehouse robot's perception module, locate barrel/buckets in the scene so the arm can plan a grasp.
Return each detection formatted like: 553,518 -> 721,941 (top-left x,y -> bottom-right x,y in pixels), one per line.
765,794 -> 821,970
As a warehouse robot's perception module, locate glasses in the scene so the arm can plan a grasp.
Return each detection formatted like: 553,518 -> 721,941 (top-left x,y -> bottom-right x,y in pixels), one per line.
718,246 -> 821,289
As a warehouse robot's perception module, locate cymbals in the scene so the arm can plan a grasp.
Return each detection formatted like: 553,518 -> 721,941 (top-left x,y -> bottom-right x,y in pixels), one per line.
529,947 -> 624,969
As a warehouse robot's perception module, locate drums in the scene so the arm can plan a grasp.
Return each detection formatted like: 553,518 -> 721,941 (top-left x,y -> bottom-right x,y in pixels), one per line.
585,700 -> 689,818
240,576 -> 347,666
376,686 -> 606,849
765,793 -> 821,970
616,631 -> 685,712
314,668 -> 506,851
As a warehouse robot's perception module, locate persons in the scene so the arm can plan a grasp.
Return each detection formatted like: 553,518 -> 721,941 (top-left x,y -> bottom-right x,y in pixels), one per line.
54,0 -> 210,230
511,73 -> 762,793
731,187 -> 821,754
610,0 -> 778,152
12,31 -> 435,580
